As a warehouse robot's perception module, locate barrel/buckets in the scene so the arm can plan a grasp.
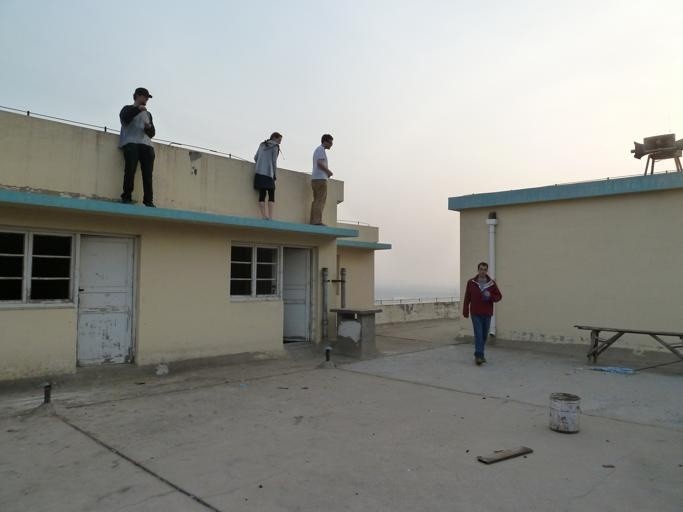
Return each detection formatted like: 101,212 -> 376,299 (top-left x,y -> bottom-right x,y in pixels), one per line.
548,392 -> 582,434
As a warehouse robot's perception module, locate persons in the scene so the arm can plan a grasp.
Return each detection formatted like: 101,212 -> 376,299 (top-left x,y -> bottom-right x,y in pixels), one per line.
117,88 -> 155,208
462,262 -> 502,366
253,132 -> 282,220
309,134 -> 332,226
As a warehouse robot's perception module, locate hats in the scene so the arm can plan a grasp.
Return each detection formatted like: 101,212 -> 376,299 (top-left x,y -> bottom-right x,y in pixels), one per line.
135,88 -> 152,98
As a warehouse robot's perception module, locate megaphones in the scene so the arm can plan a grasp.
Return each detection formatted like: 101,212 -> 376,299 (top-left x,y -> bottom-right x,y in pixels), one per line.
675,139 -> 683,152
630,141 -> 645,159
643,133 -> 676,152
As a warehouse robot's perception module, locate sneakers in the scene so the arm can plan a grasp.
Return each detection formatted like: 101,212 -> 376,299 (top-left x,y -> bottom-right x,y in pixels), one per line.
142,200 -> 155,208
475,358 -> 486,364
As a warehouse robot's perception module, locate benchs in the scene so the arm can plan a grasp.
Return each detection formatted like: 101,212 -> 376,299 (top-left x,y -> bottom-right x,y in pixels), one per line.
574,325 -> 683,371
